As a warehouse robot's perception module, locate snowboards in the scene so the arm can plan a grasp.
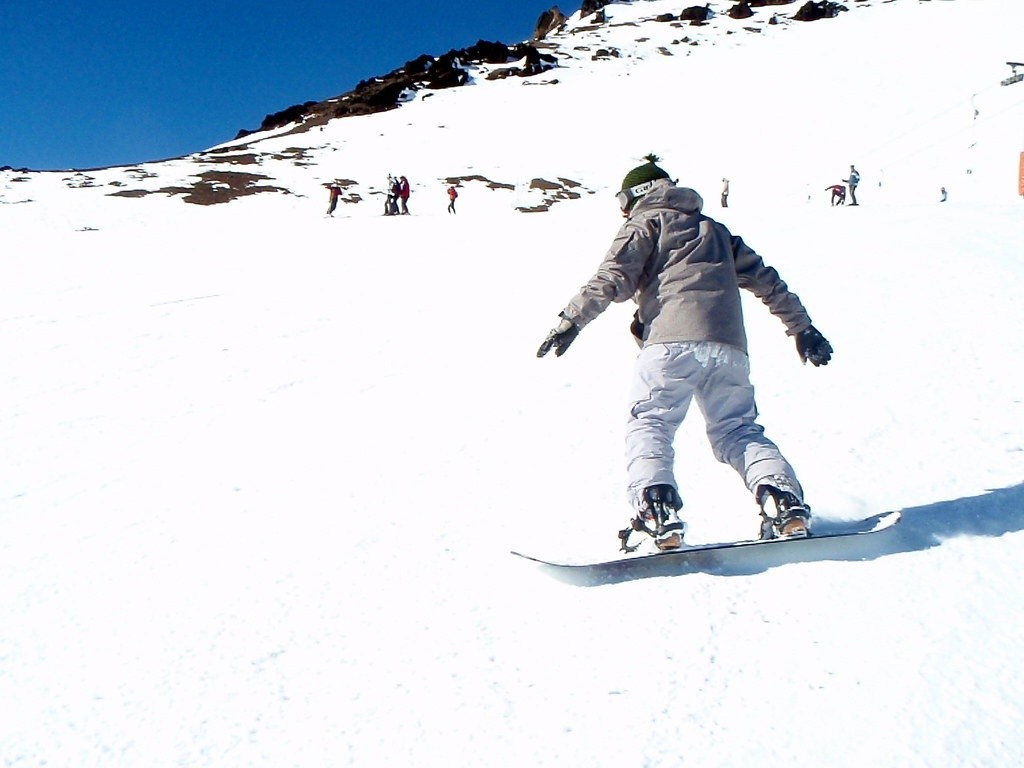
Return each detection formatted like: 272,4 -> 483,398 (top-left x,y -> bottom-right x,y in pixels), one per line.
505,504 -> 908,578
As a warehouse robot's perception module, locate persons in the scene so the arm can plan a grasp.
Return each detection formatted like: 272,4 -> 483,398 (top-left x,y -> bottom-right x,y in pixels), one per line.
842,165 -> 860,206
324,182 -> 342,214
384,176 -> 410,216
825,185 -> 845,207
447,186 -> 458,214
721,177 -> 729,207
536,153 -> 834,553
941,186 -> 947,202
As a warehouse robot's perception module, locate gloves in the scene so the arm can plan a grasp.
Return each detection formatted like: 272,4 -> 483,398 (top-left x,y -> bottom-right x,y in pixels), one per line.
793,325 -> 834,366
536,319 -> 577,358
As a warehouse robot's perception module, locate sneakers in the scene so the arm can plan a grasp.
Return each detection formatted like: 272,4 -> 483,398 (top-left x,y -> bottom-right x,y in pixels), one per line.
613,487 -> 687,551
753,489 -> 812,541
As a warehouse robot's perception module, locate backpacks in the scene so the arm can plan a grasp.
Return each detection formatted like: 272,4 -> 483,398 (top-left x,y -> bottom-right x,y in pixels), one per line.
455,191 -> 458,197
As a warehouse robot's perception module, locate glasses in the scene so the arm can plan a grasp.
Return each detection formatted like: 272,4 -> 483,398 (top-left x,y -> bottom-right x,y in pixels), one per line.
614,187 -> 634,211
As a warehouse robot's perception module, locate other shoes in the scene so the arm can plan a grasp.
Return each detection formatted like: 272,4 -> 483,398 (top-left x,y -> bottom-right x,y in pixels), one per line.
383,210 -> 408,216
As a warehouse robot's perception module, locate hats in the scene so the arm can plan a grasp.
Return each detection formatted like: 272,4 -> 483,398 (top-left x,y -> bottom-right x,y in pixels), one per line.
621,152 -> 674,210
